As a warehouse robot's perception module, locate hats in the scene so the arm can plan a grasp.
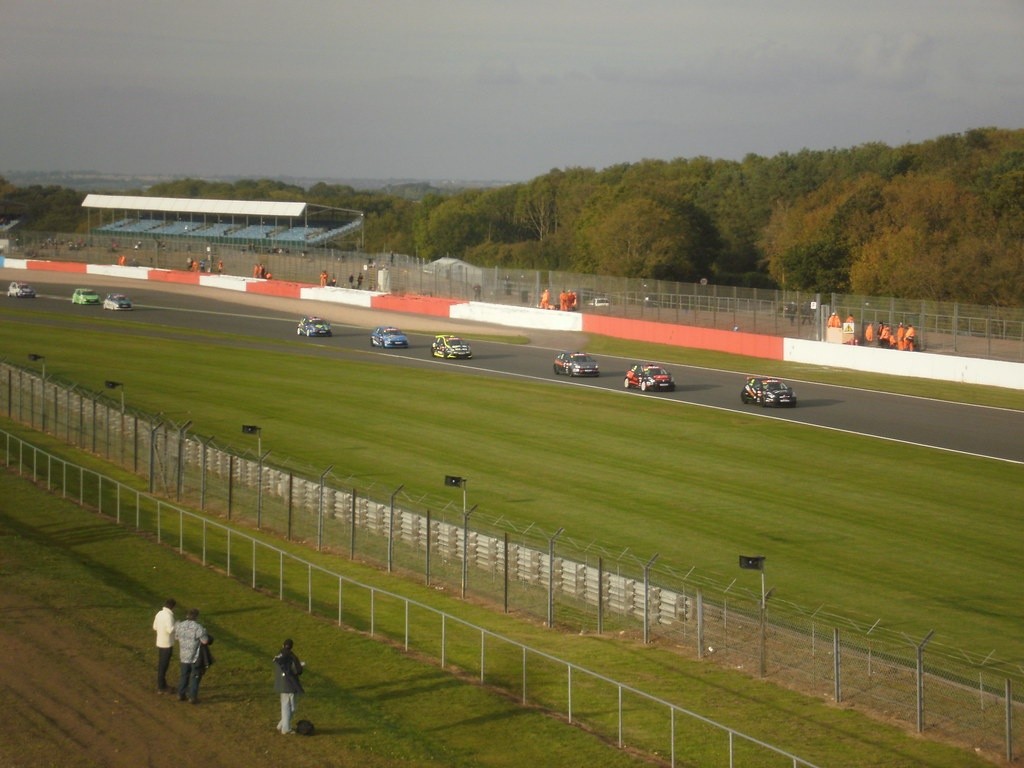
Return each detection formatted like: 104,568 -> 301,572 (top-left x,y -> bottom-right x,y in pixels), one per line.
284,639 -> 294,646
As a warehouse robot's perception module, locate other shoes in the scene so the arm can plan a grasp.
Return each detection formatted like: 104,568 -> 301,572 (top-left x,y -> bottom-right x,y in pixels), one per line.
189,698 -> 201,704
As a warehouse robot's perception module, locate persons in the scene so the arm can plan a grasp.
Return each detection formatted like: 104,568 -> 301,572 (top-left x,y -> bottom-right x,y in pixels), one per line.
175,609 -> 209,705
784,301 -> 916,352
152,600 -> 181,694
112,240 -> 577,312
275,639 -> 304,736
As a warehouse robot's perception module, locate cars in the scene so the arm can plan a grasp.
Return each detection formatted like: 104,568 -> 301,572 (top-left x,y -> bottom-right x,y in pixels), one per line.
586,297 -> 610,306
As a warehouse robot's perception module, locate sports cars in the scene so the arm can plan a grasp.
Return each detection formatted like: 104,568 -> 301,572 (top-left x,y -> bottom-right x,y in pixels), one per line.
296,316 -> 334,338
7,281 -> 36,298
101,293 -> 134,312
552,351 -> 601,378
369,326 -> 410,349
623,364 -> 677,393
71,288 -> 102,306
741,376 -> 797,409
431,335 -> 473,360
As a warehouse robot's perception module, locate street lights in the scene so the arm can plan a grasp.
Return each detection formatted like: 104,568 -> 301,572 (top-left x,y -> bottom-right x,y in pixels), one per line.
736,551 -> 770,680
444,475 -> 470,573
240,424 -> 264,509
27,353 -> 48,419
105,379 -> 126,449
